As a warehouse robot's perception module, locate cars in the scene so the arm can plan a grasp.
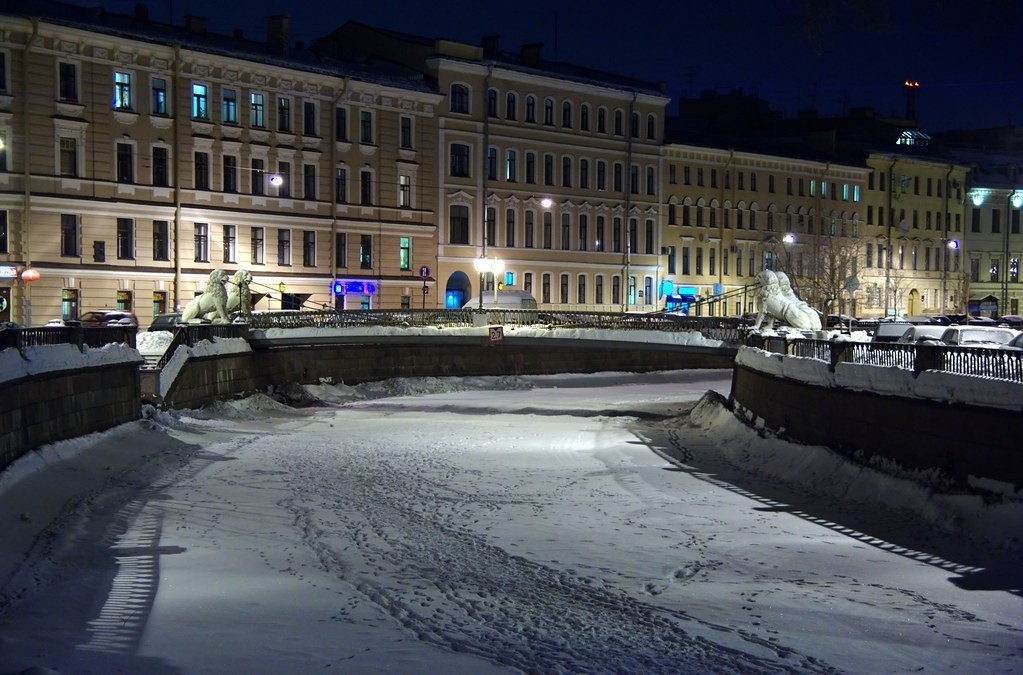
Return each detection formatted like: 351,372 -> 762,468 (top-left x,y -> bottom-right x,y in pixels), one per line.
149,313 -> 183,333
741,312 -> 1022,351
79,311 -> 137,327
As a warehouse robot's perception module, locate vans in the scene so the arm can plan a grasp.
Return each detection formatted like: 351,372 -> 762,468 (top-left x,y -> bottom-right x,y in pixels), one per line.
462,291 -> 537,324
